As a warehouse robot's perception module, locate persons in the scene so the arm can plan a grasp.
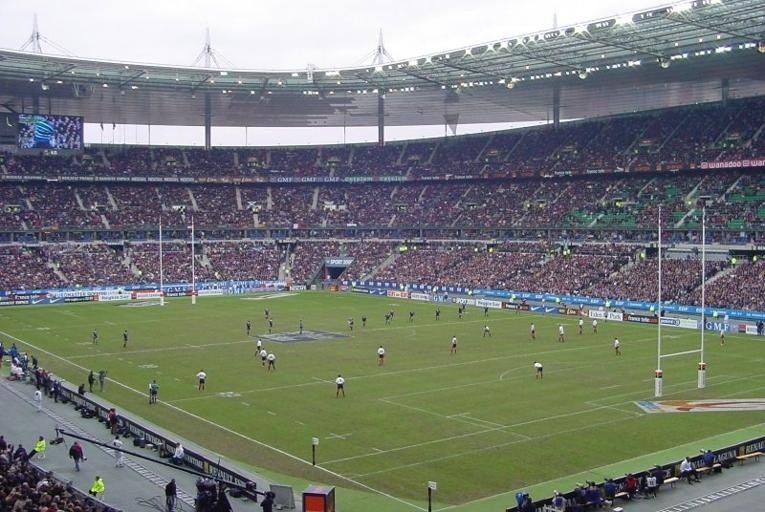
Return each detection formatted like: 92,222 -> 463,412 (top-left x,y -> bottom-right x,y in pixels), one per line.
548,448 -> 714,512
0,96 -> 765,316
1,329 -> 184,511
196,302 -> 623,398
720,328 -> 725,346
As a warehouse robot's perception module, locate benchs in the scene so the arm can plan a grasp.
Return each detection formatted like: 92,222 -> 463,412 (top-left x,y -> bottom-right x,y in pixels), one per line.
695,463 -> 722,480
586,491 -> 630,507
736,451 -> 765,466
644,476 -> 679,497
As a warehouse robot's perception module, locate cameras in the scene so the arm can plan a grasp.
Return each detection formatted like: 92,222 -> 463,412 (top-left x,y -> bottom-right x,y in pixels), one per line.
575,481 -> 584,488
699,447 -> 708,454
60,379 -> 65,382
586,480 -> 595,486
553,490 -> 561,496
